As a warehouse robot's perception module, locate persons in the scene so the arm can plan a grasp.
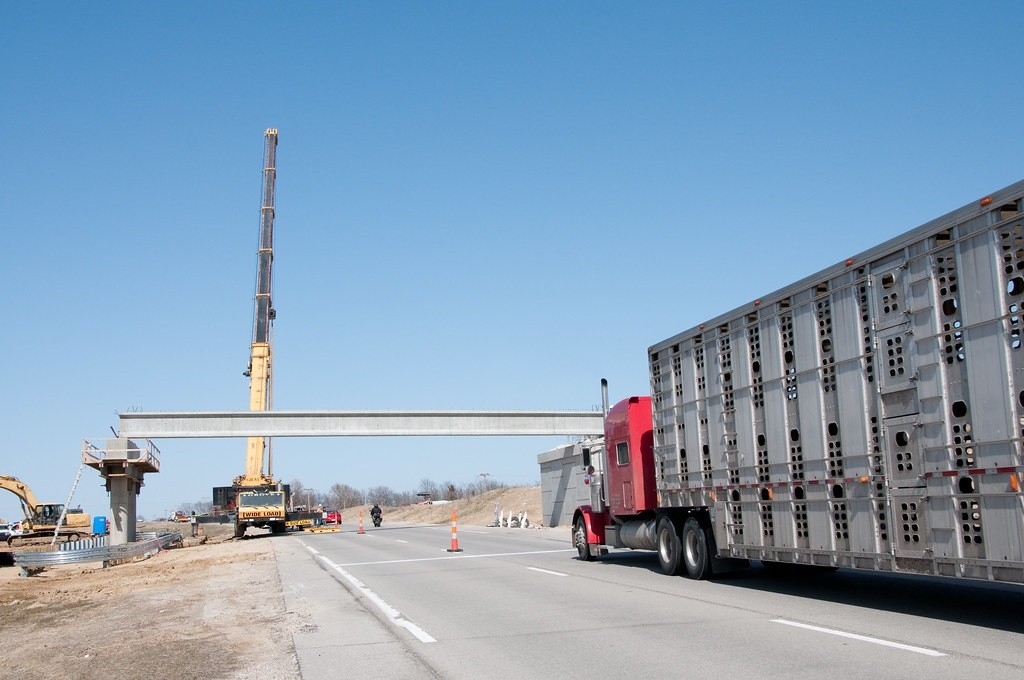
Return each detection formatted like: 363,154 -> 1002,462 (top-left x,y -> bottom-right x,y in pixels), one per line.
49,508 -> 53,521
371,504 -> 382,522
317,504 -> 326,526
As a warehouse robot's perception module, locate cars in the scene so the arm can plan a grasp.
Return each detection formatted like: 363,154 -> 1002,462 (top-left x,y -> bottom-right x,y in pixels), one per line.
0,520 -> 33,541
326,510 -> 341,524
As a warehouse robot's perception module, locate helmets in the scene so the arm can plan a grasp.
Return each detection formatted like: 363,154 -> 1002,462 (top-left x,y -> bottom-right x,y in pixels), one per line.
374,504 -> 378,507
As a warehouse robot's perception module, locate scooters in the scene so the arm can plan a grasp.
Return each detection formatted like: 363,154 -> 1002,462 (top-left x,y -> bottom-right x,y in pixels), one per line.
369,510 -> 381,527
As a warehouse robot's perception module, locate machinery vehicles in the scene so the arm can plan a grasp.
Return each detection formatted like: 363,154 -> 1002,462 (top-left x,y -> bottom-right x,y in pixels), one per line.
213,128 -> 322,537
0,474 -> 92,548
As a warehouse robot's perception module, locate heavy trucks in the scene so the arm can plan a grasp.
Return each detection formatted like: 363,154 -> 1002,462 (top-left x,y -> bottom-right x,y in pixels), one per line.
572,179 -> 1024,593
